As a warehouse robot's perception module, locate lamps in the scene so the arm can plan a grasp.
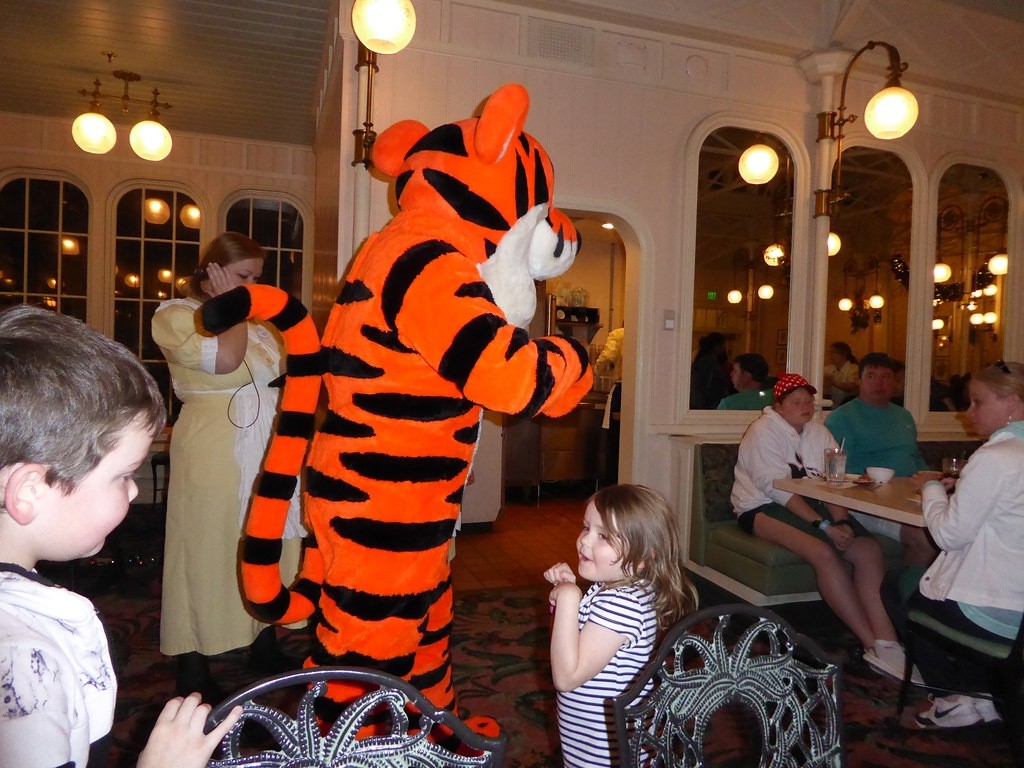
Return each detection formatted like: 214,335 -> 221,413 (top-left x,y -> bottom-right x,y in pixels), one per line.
827,229 -> 887,335
68,66 -> 176,164
115,195 -> 202,301
932,247 -> 1009,358
0,232 -> 82,308
344,0 -> 420,173
722,238 -> 787,317
811,38 -> 921,218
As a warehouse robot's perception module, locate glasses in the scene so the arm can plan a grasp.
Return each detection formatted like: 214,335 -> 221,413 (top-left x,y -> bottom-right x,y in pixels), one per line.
994,359 -> 1010,374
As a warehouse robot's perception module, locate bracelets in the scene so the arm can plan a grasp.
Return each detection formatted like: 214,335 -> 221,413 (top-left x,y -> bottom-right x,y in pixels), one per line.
812,516 -> 830,531
836,519 -> 852,528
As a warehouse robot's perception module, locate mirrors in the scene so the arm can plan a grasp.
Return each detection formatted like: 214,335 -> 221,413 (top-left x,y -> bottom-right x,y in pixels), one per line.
685,124 -> 797,415
820,145 -> 914,415
927,160 -> 1012,414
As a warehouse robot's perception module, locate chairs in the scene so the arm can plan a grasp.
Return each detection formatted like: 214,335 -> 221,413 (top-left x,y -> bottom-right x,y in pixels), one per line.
202,663 -> 507,768
607,601 -> 850,768
890,601 -> 1024,718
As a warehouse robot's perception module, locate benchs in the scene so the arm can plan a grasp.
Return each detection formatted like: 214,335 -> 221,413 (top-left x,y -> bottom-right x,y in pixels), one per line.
683,438 -> 985,609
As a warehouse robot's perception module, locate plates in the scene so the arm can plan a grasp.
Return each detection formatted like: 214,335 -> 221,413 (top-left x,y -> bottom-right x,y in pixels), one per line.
821,472 -> 881,483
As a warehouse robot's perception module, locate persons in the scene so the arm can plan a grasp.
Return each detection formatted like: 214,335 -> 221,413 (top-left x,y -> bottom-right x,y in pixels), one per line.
690,333 -> 973,411
732,374 -> 924,688
151,231 -> 309,708
907,363 -> 1024,730
544,481 -> 699,768
825,352 -> 941,569
1,300 -> 244,768
594,319 -> 625,486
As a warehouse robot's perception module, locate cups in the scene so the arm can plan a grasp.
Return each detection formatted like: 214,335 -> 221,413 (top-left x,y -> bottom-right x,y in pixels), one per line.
590,343 -> 613,394
916,471 -> 942,495
943,457 -> 963,494
825,448 -> 847,487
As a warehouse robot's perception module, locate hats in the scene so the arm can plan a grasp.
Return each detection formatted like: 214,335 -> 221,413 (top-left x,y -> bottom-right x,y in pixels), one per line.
773,374 -> 817,402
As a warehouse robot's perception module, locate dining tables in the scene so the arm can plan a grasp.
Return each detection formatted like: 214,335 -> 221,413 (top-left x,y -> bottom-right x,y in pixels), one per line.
765,473 -> 957,530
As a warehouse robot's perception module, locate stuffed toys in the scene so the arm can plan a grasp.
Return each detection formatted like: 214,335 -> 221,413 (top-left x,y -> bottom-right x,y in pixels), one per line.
191,80 -> 595,759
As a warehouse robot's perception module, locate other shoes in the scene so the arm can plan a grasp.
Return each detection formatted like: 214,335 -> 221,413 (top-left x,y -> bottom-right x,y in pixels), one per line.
176,653 -> 226,702
250,627 -> 300,671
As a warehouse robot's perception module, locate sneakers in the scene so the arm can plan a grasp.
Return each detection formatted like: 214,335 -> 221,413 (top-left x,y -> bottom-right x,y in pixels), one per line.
914,691 -> 1003,729
862,639 -> 924,688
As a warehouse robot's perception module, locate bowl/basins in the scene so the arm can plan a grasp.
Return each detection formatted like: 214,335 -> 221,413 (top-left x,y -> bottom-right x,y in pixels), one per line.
866,466 -> 895,484
